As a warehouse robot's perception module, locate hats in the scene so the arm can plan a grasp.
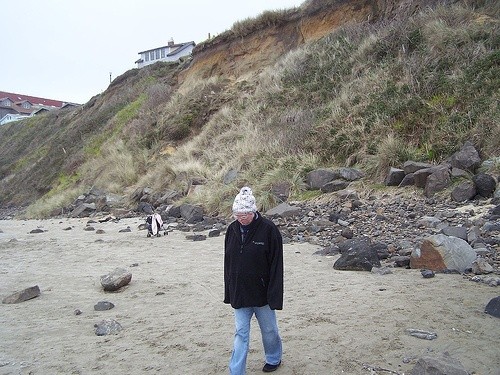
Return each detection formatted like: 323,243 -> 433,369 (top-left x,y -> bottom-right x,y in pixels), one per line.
231,187 -> 256,213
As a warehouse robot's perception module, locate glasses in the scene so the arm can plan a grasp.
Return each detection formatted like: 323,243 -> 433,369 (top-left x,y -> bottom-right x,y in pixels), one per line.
234,214 -> 250,219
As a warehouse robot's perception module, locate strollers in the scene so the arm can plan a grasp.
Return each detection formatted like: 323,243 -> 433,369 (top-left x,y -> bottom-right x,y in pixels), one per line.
145,215 -> 168,237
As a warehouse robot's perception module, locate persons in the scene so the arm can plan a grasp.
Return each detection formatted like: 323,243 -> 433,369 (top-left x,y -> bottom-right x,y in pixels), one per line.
223,186 -> 283,375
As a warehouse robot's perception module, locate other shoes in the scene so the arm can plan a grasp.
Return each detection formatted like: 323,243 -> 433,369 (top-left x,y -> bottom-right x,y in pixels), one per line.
263,360 -> 282,372
